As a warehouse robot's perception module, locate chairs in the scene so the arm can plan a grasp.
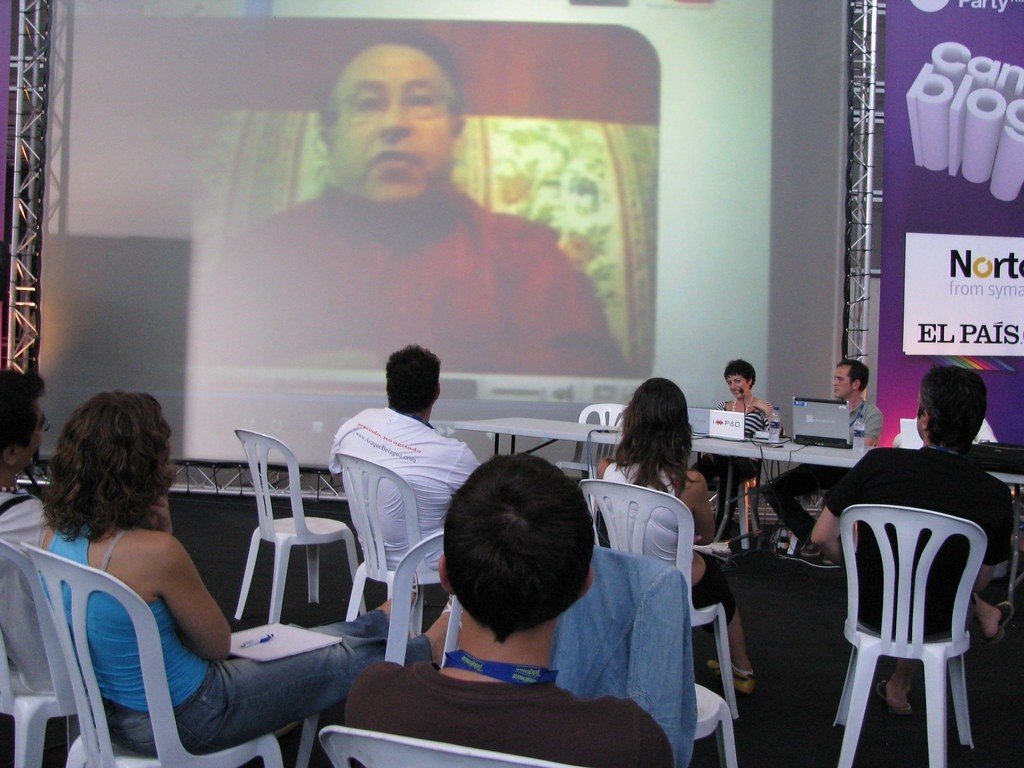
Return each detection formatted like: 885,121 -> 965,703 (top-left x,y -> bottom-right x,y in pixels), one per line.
233,429 -> 366,624
318,723 -> 587,768
385,529 -> 462,670
711,477 -> 757,550
830,502 -> 988,768
786,490 -> 858,556
556,403 -> 630,475
333,451 -> 444,638
559,548 -> 738,768
19,541 -> 322,768
0,541 -> 76,768
579,478 -> 739,721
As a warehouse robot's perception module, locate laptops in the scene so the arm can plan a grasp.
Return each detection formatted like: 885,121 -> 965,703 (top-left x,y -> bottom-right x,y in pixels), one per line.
900,419 -> 924,450
709,410 -> 752,442
792,397 -> 854,450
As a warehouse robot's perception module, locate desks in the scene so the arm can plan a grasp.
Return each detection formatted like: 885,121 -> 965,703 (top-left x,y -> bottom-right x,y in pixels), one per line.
451,417 -> 1024,517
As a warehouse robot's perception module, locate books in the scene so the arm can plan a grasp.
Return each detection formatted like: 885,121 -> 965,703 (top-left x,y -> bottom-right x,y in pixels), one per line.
229,623 -> 342,661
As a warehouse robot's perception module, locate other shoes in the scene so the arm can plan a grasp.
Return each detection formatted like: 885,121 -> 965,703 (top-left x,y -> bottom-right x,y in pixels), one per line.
801,543 -> 822,558
823,555 -> 840,566
714,512 -> 735,540
707,660 -> 757,694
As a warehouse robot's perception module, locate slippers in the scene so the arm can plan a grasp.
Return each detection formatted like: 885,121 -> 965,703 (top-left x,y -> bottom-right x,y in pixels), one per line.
877,680 -> 913,715
980,600 -> 1015,648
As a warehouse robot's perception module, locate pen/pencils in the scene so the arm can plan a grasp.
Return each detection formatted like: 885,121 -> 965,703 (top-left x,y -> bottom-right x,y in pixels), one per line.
240,633 -> 274,648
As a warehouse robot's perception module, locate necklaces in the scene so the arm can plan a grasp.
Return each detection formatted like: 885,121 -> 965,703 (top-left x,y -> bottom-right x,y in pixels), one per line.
732,395 -> 753,413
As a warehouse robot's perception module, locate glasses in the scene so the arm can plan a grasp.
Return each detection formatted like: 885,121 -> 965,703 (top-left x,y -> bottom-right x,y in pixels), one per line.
325,78 -> 463,125
31,417 -> 50,432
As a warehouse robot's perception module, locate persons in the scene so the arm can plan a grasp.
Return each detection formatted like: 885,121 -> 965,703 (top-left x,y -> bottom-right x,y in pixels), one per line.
690,360 -> 774,536
596,377 -> 757,693
345,453 -> 674,768
764,360 -> 884,564
199,39 -> 630,376
39,389 -> 463,756
329,344 -> 478,580
0,370 -> 51,694
810,369 -> 1015,714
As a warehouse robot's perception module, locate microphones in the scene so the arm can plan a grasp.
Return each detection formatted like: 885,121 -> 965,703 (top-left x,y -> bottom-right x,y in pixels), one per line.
739,388 -> 753,439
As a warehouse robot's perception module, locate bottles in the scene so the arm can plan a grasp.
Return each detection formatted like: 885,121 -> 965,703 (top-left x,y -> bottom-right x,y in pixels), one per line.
768,406 -> 781,442
852,414 -> 865,452
